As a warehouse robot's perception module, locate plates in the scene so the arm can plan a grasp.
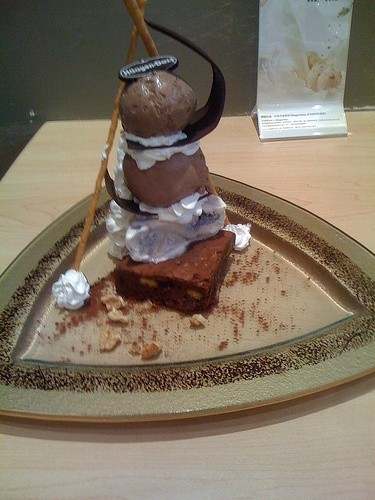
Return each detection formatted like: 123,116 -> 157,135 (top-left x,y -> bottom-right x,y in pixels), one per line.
0,173 -> 374,424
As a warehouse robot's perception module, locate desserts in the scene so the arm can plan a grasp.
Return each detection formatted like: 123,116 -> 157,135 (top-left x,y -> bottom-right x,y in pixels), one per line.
51,0 -> 252,315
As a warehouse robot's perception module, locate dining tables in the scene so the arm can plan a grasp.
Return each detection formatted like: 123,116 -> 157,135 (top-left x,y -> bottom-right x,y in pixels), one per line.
1,109 -> 375,500
